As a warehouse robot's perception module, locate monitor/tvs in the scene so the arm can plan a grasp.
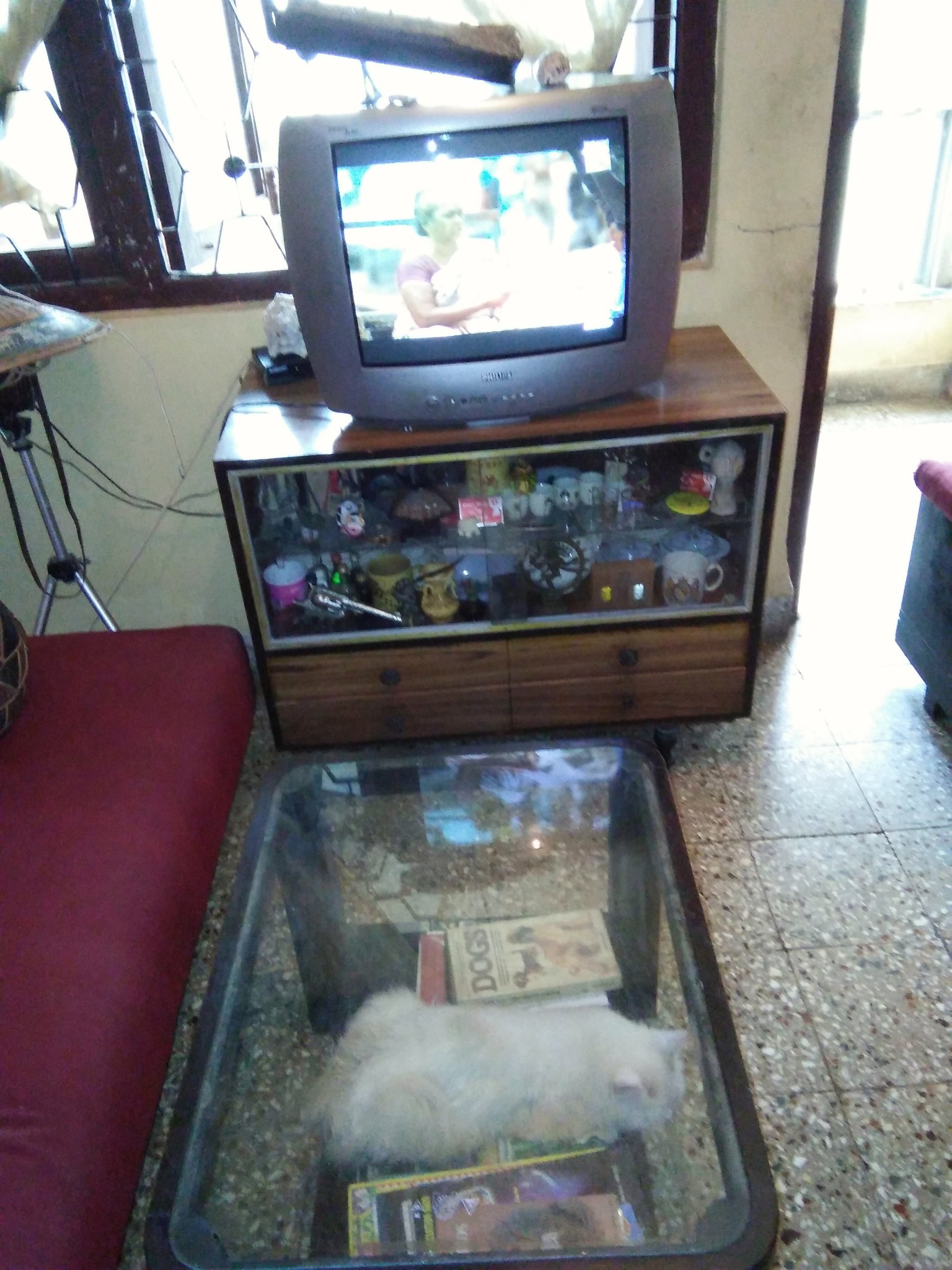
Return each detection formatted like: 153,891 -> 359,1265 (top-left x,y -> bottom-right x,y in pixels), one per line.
278,75 -> 683,430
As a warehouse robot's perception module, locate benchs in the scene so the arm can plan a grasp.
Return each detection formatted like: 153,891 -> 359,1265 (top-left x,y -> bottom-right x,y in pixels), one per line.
0,627 -> 255,1270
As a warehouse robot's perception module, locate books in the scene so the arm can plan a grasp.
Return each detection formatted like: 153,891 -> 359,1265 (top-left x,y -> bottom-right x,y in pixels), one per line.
417,908 -> 624,1005
347,1145 -> 644,1256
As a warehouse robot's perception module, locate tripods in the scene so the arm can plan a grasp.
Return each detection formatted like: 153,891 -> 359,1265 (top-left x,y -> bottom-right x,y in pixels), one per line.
3,417 -> 119,637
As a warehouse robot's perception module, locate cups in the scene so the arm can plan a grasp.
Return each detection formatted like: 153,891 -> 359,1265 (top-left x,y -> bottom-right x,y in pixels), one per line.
264,464 -> 725,635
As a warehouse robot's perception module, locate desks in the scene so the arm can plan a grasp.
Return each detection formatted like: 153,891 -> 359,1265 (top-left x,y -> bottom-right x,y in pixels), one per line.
150,730 -> 780,1270
0,295 -> 119,636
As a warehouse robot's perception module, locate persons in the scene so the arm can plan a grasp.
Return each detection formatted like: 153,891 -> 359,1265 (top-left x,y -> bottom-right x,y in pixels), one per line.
397,180 -> 509,337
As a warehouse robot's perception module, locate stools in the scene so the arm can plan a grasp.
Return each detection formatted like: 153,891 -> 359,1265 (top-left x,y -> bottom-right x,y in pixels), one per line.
895,461 -> 952,722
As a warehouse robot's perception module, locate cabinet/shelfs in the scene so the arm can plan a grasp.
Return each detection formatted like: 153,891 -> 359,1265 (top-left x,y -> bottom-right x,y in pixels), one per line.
211,324 -> 789,751
